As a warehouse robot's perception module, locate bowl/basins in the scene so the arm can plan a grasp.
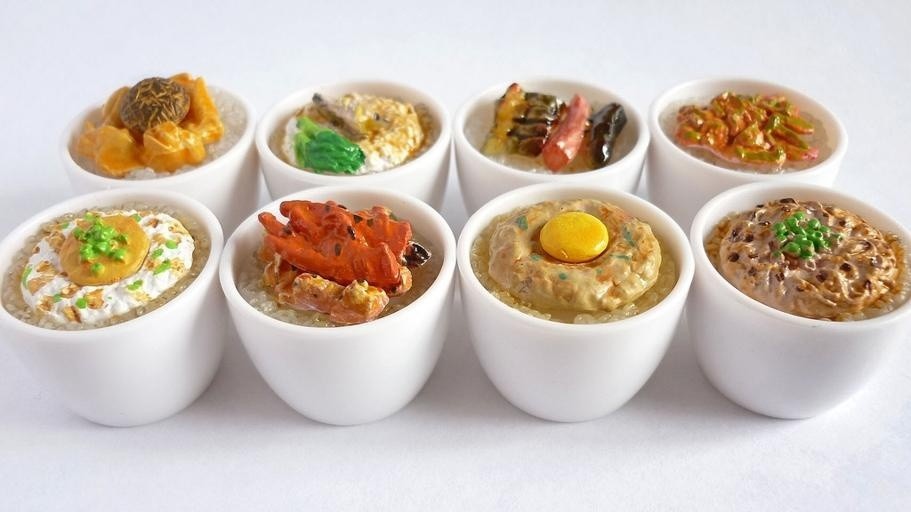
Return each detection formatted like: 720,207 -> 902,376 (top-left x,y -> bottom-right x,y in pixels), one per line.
453,76 -> 649,228
220,182 -> 458,426
458,181 -> 696,423
61,78 -> 254,228
649,75 -> 848,241
690,181 -> 911,421
1,187 -> 223,428
255,77 -> 453,234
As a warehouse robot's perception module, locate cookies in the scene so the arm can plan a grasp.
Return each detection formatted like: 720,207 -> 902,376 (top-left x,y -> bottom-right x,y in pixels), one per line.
488,196 -> 663,309
717,198 -> 898,320
19,210 -> 193,322
282,93 -> 423,176
76,72 -> 223,177
